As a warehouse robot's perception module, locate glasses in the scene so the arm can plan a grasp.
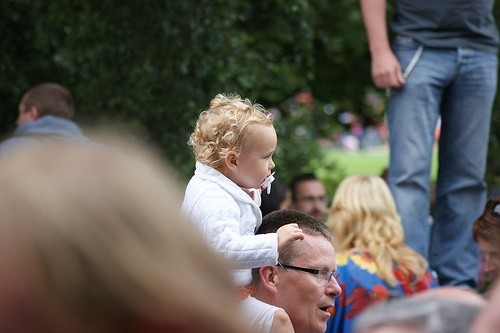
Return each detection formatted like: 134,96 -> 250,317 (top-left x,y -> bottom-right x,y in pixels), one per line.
276,262 -> 341,285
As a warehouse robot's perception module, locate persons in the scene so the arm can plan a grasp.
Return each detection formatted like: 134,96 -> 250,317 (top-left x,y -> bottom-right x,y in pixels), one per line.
361,0 -> 500,294
323,175 -> 438,332
1,134 -> 249,333
293,88 -> 441,156
473,201 -> 500,304
1,82 -> 123,168
351,285 -> 488,333
179,91 -> 305,333
288,173 -> 327,221
259,176 -> 294,218
251,207 -> 342,333
